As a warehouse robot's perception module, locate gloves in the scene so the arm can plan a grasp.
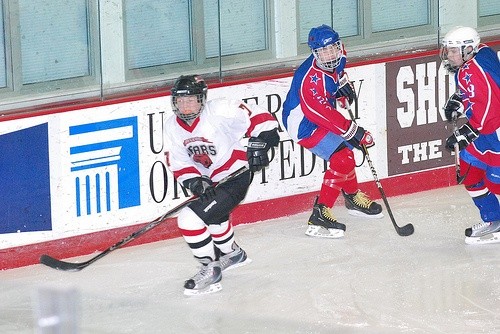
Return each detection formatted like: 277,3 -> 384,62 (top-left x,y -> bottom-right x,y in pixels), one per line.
183,174 -> 217,204
445,122 -> 479,154
336,72 -> 354,109
340,120 -> 375,153
245,135 -> 270,171
443,93 -> 466,123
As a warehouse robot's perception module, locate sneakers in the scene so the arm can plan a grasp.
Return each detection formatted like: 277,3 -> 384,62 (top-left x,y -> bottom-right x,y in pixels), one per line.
305,195 -> 346,238
219,241 -> 252,272
183,247 -> 222,295
341,189 -> 384,218
464,220 -> 500,243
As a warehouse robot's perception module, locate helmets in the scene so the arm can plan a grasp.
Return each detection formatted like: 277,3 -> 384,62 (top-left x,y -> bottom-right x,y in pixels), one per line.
439,25 -> 480,74
170,74 -> 208,121
307,24 -> 343,70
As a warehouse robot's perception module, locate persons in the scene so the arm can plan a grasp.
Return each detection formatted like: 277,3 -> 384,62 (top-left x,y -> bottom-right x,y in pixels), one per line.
162,74 -> 279,295
439,26 -> 500,244
282,24 -> 385,239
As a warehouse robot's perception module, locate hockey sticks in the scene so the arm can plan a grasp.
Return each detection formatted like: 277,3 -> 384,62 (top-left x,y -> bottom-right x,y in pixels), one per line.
345,98 -> 414,236
452,110 -> 472,186
39,163 -> 250,273
